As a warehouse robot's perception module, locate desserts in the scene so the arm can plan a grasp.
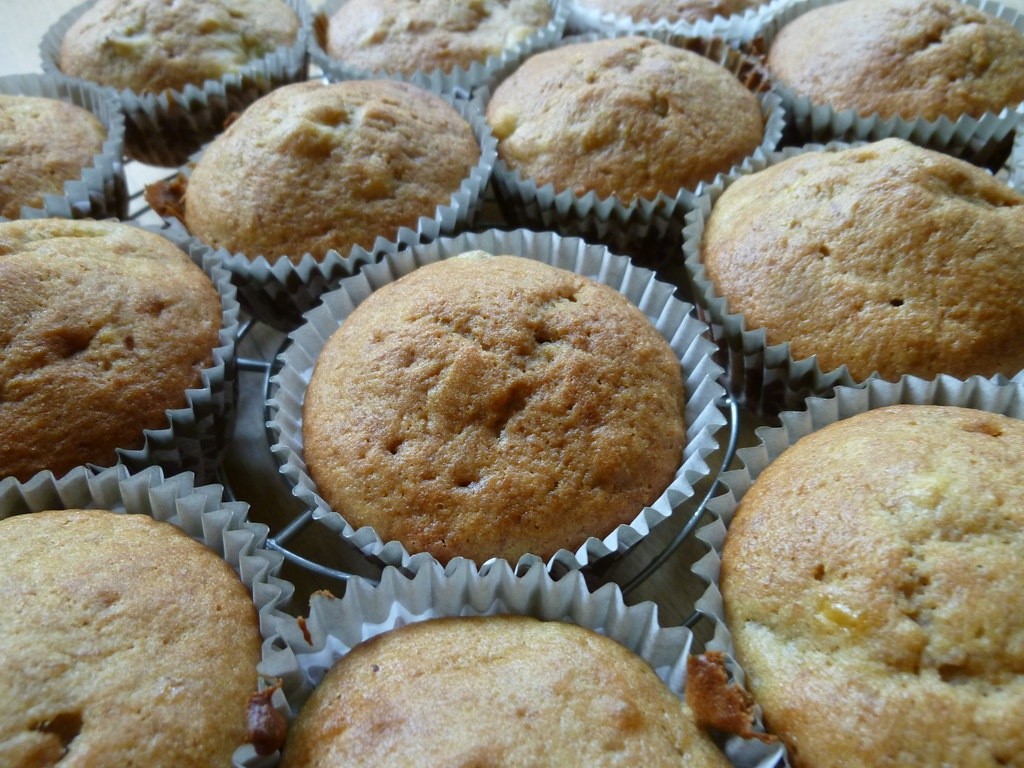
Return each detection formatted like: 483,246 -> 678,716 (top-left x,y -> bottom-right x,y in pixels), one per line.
0,0 -> 1024,767
